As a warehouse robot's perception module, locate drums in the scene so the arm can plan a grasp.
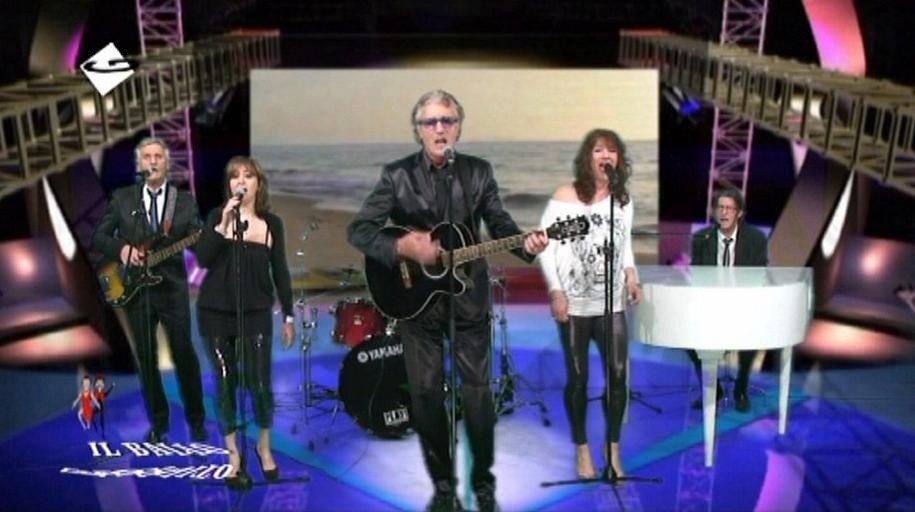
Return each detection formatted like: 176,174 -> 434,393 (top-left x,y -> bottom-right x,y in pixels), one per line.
337,336 -> 414,437
332,299 -> 392,347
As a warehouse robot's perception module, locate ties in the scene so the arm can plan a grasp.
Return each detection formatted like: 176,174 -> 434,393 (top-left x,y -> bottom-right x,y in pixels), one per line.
721,235 -> 734,268
432,166 -> 449,223
147,188 -> 162,234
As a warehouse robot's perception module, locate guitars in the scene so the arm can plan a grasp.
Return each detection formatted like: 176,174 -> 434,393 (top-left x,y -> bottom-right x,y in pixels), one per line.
96,234 -> 205,306
366,218 -> 589,322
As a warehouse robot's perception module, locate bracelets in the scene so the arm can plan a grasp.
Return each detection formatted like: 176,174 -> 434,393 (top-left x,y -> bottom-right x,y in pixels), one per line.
283,315 -> 294,322
551,296 -> 566,301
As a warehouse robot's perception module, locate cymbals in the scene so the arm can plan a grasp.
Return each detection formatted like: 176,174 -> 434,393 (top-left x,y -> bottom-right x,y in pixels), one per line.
310,264 -> 363,284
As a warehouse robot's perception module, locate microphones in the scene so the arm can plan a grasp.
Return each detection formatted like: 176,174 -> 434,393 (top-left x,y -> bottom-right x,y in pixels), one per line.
604,163 -> 618,185
705,223 -> 720,239
135,165 -> 158,176
233,187 -> 246,213
443,146 -> 456,164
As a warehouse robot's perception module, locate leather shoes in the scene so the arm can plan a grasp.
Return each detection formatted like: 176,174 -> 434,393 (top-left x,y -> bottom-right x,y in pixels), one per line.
253,445 -> 280,481
224,456 -> 246,482
147,424 -> 170,445
733,382 -> 750,411
692,382 -> 725,408
604,452 -> 623,480
576,454 -> 594,480
190,428 -> 209,442
474,484 -> 496,512
426,480 -> 464,512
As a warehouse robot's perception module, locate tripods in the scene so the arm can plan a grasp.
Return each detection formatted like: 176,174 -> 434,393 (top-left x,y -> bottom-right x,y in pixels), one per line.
541,185 -> 664,512
77,178 -> 208,475
456,286 -> 551,426
273,296 -> 341,450
190,210 -> 310,512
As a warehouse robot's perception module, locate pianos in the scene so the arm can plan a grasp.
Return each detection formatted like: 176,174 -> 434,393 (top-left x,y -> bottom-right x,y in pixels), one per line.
627,265 -> 813,467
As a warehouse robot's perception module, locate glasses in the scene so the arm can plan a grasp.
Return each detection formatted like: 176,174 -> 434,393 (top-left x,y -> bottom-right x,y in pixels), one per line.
716,205 -> 740,215
417,117 -> 458,133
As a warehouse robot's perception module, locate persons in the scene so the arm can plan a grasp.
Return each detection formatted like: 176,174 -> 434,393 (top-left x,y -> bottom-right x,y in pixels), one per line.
92,377 -> 116,439
72,376 -> 101,430
346,90 -> 549,512
686,190 -> 766,411
537,130 -> 642,479
94,138 -> 208,441
196,156 -> 294,483
